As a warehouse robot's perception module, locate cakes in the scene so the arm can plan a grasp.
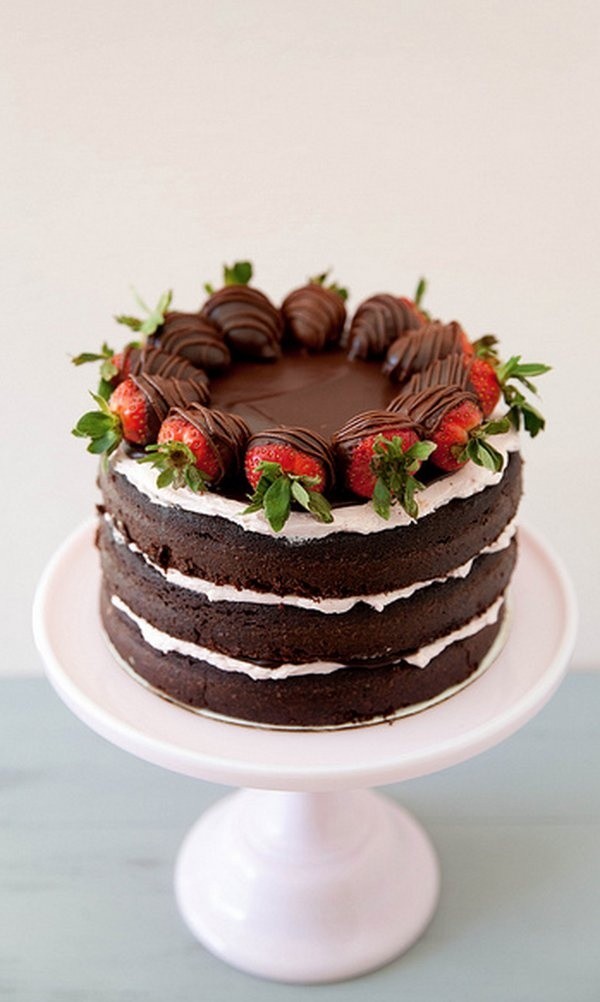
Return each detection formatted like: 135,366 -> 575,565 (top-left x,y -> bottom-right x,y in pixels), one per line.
67,262 -> 548,729
